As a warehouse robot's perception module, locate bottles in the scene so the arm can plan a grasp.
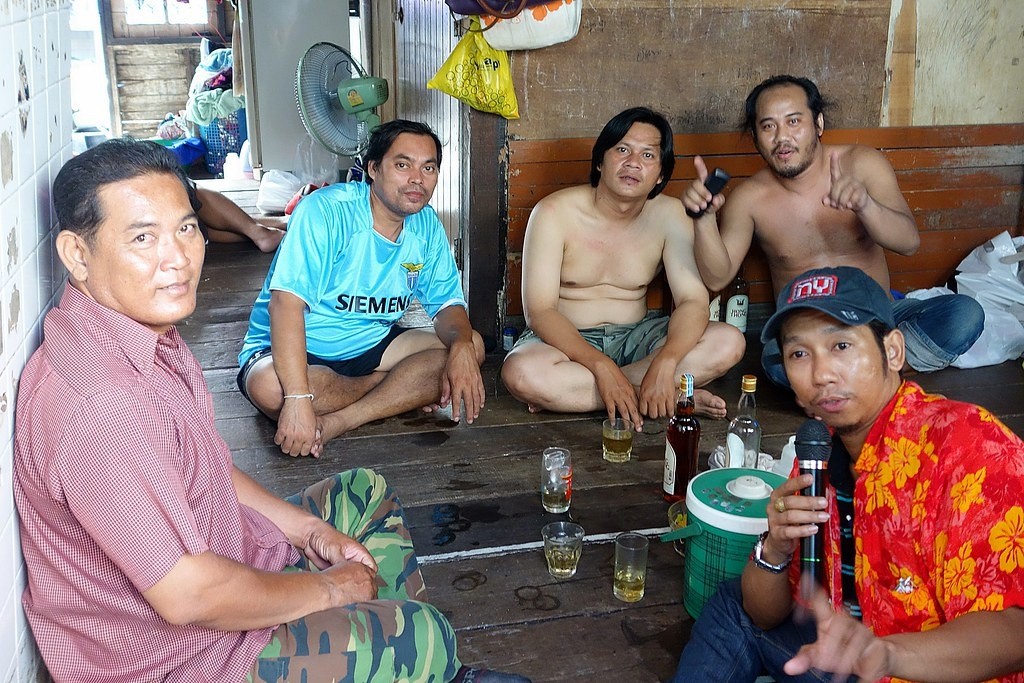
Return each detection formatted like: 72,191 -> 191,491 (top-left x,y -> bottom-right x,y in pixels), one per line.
723,258 -> 751,340
724,374 -> 763,469
663,373 -> 699,506
780,435 -> 795,479
503,327 -> 518,352
705,287 -> 721,327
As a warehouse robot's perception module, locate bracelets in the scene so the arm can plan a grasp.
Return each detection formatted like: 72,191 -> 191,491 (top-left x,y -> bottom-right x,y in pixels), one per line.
283,393 -> 314,400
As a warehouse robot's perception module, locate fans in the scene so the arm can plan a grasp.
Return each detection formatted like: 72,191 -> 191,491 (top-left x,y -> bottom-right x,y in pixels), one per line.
295,42 -> 388,156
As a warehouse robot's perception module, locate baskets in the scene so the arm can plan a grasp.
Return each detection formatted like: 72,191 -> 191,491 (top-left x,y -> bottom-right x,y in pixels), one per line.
198,108 -> 243,173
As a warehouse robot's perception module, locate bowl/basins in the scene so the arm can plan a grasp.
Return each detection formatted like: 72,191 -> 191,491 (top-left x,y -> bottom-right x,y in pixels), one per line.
667,500 -> 687,555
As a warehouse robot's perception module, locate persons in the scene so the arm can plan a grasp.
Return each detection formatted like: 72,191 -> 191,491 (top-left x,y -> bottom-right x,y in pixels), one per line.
234,117 -> 486,458
501,107 -> 751,433
192,179 -> 292,251
679,75 -> 986,389
671,264 -> 1024,683
14,136 -> 532,683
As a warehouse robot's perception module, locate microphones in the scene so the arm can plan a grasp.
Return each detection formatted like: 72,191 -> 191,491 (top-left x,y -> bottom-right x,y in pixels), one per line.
793,418 -> 833,586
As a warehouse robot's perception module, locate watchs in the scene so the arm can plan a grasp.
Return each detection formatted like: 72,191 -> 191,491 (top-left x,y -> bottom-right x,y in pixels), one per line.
752,531 -> 794,574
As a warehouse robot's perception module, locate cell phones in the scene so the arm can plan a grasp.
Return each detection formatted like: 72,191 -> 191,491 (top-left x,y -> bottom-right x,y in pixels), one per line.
686,168 -> 732,218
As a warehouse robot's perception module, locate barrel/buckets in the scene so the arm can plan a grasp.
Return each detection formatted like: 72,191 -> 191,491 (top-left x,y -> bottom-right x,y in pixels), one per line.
659,467 -> 790,621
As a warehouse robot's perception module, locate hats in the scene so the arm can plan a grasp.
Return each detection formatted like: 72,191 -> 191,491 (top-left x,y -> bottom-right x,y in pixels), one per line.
762,267 -> 897,342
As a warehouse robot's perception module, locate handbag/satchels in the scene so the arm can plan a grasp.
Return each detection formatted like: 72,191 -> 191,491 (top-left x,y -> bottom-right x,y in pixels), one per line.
445,0 -> 552,31
480,0 -> 581,51
428,16 -> 519,121
904,231 -> 1024,369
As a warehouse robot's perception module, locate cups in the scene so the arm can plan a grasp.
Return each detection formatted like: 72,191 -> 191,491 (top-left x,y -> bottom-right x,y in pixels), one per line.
602,418 -> 635,462
542,521 -> 586,578
613,532 -> 649,602
540,448 -> 572,513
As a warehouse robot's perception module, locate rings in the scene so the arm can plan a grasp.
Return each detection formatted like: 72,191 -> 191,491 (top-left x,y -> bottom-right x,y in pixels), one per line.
774,497 -> 787,512
